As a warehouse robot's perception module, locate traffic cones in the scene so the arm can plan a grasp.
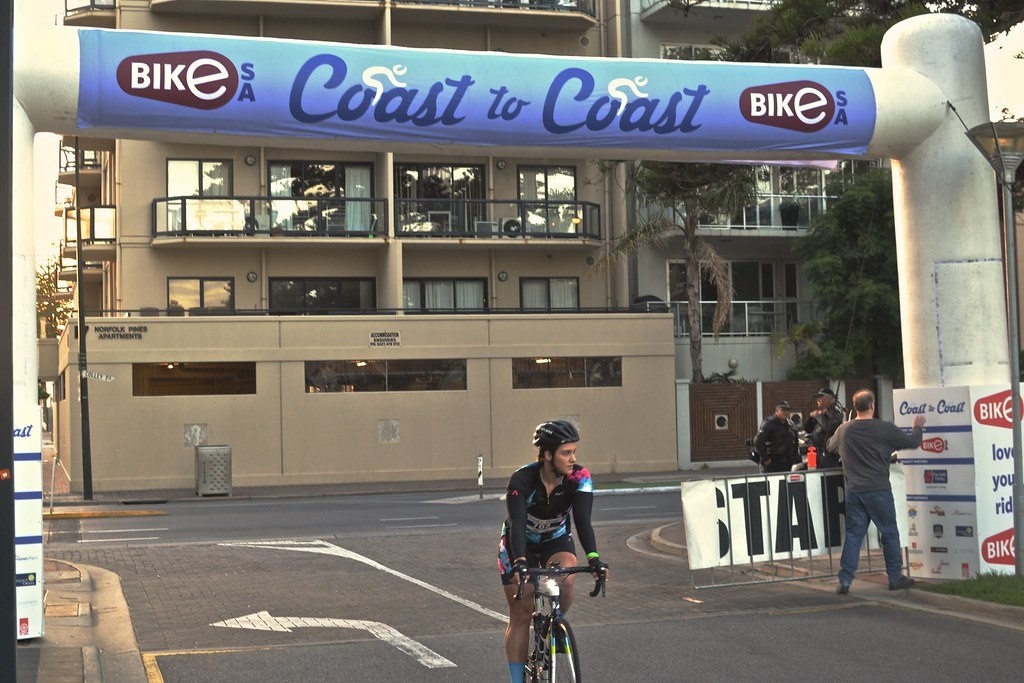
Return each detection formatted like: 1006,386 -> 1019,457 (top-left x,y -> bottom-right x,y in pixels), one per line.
805,446 -> 819,470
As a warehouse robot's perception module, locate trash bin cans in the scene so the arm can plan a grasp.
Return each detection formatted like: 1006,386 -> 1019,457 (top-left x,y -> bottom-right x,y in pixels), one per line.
194,444 -> 233,498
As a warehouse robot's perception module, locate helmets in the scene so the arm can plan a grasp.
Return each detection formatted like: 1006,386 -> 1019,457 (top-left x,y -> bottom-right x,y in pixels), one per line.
532,420 -> 580,447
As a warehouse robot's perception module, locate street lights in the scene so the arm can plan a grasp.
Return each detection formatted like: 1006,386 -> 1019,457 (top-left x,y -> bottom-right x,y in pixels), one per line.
966,117 -> 1024,588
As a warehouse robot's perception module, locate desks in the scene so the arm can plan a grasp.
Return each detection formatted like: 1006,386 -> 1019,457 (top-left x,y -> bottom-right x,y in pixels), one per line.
426,211 -> 452,239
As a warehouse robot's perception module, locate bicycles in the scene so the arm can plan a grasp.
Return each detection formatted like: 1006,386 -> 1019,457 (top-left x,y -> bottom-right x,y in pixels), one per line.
512,561 -> 609,682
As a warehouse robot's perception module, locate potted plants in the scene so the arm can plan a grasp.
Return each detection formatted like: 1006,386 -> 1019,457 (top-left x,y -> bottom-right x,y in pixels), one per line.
780,203 -> 799,230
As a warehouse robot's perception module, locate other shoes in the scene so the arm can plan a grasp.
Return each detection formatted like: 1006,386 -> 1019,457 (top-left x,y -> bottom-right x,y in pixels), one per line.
889,575 -> 915,590
554,627 -> 571,653
836,585 -> 849,594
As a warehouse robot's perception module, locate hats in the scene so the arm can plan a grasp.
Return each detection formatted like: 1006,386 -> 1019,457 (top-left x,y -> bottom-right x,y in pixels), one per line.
777,401 -> 793,410
813,388 -> 834,399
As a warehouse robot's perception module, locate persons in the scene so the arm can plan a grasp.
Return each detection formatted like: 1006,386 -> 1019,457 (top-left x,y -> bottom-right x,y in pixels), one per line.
804,388 -> 843,469
826,389 -> 927,591
752,401 -> 797,473
498,420 -> 609,683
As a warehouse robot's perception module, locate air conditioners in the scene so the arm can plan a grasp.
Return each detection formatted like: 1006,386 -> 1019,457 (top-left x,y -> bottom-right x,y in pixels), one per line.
474,221 -> 498,238
498,218 -> 531,239
